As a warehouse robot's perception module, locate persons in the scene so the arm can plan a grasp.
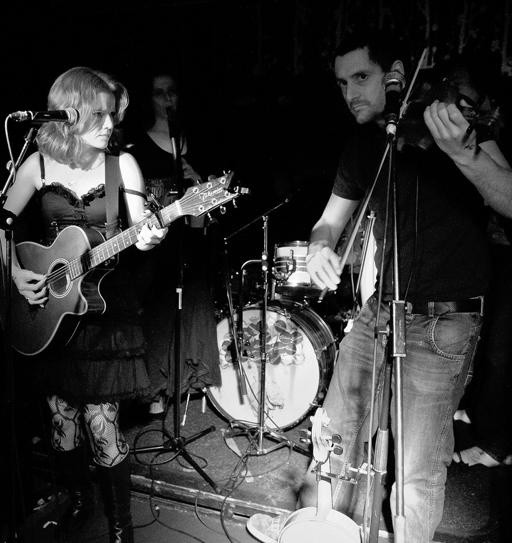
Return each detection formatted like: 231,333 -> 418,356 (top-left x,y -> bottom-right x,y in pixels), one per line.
123,70 -> 203,417
0,65 -> 167,543
297,32 -> 512,543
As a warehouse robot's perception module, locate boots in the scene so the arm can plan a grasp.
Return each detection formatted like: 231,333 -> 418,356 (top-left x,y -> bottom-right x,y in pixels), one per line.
64,472 -> 134,542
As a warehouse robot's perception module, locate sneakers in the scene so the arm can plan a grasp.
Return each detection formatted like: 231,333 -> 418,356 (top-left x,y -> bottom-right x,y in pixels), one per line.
247,513 -> 287,542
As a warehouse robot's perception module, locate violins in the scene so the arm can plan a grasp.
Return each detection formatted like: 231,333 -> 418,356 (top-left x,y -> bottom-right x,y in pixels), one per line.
369,51 -> 510,150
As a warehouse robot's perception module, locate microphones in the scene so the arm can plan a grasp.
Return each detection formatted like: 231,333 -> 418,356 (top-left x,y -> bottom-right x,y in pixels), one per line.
235,357 -> 247,396
9,107 -> 80,126
382,72 -> 405,137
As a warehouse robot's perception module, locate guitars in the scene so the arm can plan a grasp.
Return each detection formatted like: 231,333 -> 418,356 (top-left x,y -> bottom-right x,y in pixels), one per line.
1,168 -> 249,357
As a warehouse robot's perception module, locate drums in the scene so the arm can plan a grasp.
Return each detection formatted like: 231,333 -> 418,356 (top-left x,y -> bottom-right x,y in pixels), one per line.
273,240 -> 332,296
201,297 -> 335,432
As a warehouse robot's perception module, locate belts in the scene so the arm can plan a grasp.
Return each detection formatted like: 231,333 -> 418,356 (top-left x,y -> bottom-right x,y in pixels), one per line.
404,300 -> 483,316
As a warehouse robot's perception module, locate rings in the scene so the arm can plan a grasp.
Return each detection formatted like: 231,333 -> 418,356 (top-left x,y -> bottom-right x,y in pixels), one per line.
26,298 -> 29,300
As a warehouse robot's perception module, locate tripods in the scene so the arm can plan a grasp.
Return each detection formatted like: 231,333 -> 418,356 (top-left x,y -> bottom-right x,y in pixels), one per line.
129,247 -> 224,496
226,198 -> 313,492
